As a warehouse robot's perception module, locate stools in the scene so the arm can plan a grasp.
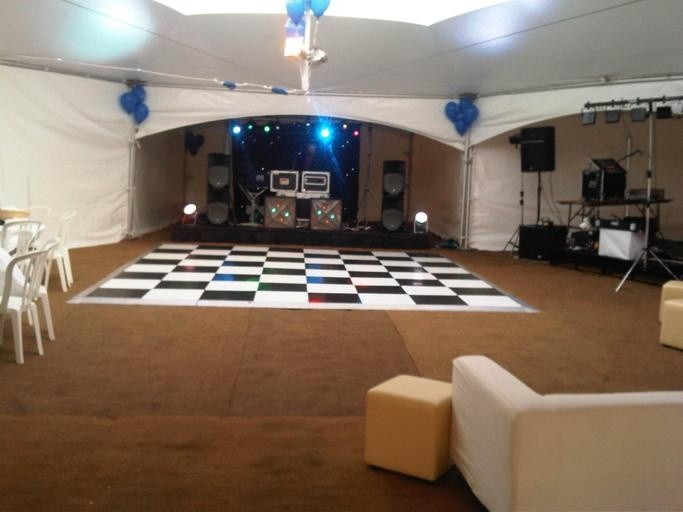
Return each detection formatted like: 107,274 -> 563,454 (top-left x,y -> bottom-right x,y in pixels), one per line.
363,373 -> 455,485
657,296 -> 683,353
656,278 -> 683,322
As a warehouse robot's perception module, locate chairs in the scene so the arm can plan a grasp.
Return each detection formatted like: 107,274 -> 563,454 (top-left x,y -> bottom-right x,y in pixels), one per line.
0,203 -> 79,366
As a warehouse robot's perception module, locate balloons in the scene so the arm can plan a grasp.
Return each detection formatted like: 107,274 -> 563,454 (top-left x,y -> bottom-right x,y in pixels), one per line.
286,0 -> 307,27
308,0 -> 331,22
445,96 -> 479,137
131,103 -> 149,125
120,93 -> 138,115
131,86 -> 145,103
185,131 -> 204,157
285,17 -> 305,40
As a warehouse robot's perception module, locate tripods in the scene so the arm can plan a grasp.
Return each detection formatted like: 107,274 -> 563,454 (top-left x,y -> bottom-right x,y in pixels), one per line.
613,122 -> 681,292
502,173 -> 531,255
357,134 -> 382,228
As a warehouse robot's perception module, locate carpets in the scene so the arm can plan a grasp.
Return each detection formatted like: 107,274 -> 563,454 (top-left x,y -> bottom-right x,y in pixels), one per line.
66,240 -> 544,314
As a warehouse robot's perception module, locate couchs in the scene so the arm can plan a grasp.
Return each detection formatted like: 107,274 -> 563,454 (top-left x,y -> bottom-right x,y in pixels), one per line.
447,350 -> 683,511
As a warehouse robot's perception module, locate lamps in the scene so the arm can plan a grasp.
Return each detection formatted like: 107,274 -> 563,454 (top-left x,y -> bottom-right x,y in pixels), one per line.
179,200 -> 201,227
284,0 -> 332,98
577,92 -> 682,129
407,209 -> 429,235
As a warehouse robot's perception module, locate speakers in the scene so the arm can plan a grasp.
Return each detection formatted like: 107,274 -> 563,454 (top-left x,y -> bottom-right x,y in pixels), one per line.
379,159 -> 406,232
521,126 -> 555,172
206,153 -> 231,226
